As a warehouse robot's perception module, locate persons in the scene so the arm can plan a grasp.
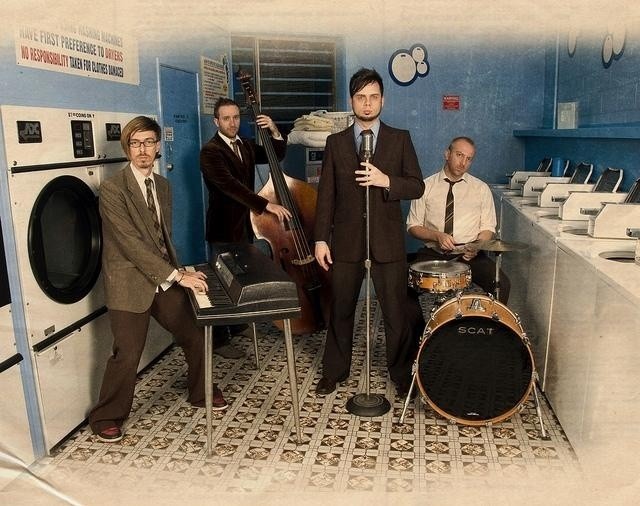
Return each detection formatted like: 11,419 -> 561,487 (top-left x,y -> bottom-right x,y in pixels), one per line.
396,136 -> 512,351
198,97 -> 295,361
311,67 -> 426,400
86,114 -> 231,444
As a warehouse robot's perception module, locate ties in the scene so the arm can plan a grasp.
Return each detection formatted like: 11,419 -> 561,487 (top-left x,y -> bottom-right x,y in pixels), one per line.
144,178 -> 172,266
444,179 -> 464,241
230,141 -> 241,165
359,128 -> 373,165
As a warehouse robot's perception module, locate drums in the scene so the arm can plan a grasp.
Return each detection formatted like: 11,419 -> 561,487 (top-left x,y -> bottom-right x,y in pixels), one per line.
409,261 -> 473,294
416,294 -> 536,427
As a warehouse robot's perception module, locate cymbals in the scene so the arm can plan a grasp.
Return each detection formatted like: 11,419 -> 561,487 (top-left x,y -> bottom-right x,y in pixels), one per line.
470,239 -> 529,252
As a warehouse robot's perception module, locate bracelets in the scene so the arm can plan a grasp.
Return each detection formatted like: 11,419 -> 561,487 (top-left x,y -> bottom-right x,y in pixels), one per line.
178,272 -> 184,284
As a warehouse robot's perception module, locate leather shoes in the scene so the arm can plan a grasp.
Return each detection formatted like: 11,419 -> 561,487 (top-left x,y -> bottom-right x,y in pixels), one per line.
397,384 -> 419,402
213,343 -> 246,359
231,326 -> 267,339
315,372 -> 347,395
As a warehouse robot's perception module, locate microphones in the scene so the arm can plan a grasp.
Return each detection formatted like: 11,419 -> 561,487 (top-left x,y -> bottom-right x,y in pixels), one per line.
361,129 -> 373,157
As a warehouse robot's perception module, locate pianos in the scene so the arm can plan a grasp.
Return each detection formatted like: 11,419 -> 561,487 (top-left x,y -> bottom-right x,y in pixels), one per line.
177,243 -> 301,326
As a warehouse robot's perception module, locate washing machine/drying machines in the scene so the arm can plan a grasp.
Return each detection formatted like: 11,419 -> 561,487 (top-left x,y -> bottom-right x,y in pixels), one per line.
0,102 -> 175,493
485,157 -> 640,506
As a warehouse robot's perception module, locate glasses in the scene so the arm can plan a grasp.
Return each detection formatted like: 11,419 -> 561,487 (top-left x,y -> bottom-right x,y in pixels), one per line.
127,137 -> 160,147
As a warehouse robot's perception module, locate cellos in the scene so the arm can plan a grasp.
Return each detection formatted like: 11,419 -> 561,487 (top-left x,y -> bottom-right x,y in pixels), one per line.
236,66 -> 333,336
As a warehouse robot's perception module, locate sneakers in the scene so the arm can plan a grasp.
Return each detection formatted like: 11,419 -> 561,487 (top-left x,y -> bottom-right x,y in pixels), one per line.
96,425 -> 123,443
191,394 -> 230,411
